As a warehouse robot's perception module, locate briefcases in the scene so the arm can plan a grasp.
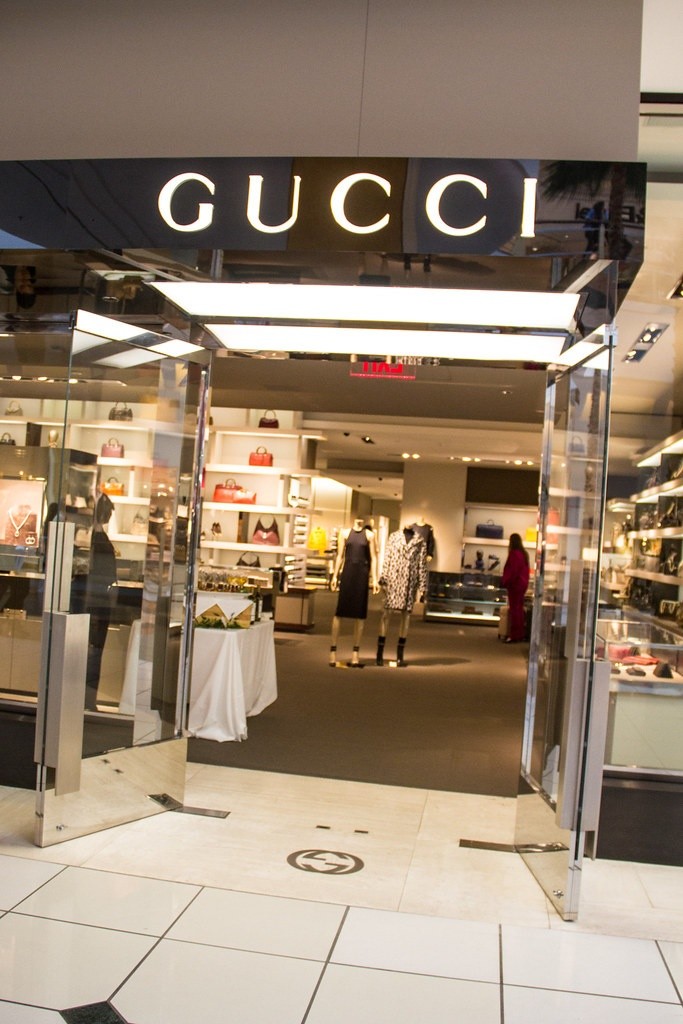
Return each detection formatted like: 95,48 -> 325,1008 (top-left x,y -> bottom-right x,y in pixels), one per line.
476,519 -> 504,539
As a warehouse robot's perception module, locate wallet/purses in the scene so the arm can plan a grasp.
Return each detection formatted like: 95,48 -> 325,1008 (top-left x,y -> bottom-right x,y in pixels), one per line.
626,667 -> 647,676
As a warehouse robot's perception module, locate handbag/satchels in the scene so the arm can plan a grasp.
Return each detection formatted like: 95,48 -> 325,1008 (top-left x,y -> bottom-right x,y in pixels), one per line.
108,400 -> 133,422
0,432 -> 16,446
116,568 -> 130,581
249,446 -> 273,467
100,437 -> 124,458
259,410 -> 279,428
99,476 -> 125,496
4,400 -> 23,416
232,488 -> 257,504
212,478 -> 243,503
253,514 -> 280,545
237,552 -> 261,567
133,505 -> 149,524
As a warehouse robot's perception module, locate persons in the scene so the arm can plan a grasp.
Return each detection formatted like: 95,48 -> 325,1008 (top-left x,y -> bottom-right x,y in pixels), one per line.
501,532 -> 530,644
84,493 -> 118,714
328,518 -> 382,668
376,515 -> 435,665
86,495 -> 95,510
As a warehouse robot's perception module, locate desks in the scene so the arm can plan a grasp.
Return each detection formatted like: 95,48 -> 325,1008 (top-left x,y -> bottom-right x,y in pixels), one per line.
118,620 -> 278,740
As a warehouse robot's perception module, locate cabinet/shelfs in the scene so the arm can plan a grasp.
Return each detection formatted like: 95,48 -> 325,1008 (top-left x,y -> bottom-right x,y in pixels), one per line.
0,396 -> 328,705
424,430 -> 682,792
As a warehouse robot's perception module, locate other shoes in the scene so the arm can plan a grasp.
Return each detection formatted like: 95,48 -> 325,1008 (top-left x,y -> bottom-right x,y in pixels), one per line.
503,637 -> 516,643
212,522 -> 222,534
493,608 -> 500,616
488,554 -> 499,560
463,606 -> 475,613
488,561 -> 500,571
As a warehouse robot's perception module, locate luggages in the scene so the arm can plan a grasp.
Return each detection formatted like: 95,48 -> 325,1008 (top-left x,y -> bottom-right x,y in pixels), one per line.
497,596 -> 510,640
